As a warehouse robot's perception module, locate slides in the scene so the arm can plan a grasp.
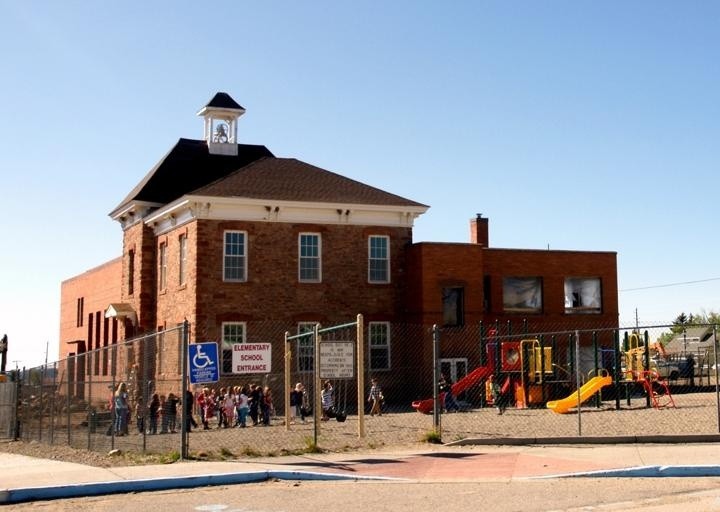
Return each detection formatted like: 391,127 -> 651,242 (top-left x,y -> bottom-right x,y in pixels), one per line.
412,367 -> 492,413
547,377 -> 612,413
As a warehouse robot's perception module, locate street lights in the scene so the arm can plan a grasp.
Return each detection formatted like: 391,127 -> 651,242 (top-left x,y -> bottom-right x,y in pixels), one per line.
1,335 -> 8,376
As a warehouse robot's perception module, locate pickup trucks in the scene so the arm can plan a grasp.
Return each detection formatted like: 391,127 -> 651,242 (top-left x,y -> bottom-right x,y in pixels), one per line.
621,360 -> 680,380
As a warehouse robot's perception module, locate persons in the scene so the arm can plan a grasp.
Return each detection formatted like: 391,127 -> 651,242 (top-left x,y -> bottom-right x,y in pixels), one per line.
489,374 -> 506,414
107,379 -> 339,436
438,372 -> 459,413
368,377 -> 384,416
